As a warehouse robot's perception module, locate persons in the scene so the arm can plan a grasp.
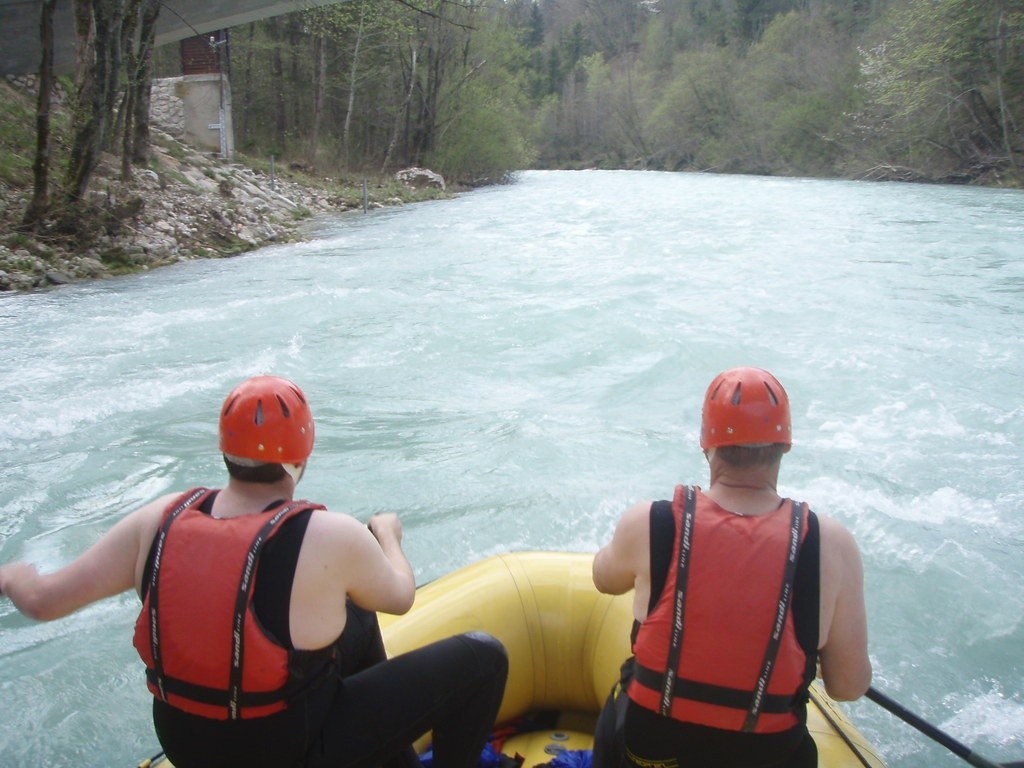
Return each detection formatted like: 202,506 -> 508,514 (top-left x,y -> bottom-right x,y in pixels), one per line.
0,375 -> 521,768
593,368 -> 873,768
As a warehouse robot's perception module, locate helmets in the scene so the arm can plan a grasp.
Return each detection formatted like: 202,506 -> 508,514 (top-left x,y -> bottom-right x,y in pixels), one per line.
701,372 -> 791,453
219,382 -> 315,464
